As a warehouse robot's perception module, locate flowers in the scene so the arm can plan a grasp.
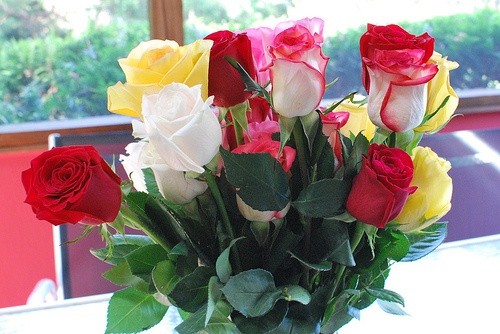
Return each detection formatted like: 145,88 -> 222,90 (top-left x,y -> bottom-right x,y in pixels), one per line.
27,21 -> 466,334
